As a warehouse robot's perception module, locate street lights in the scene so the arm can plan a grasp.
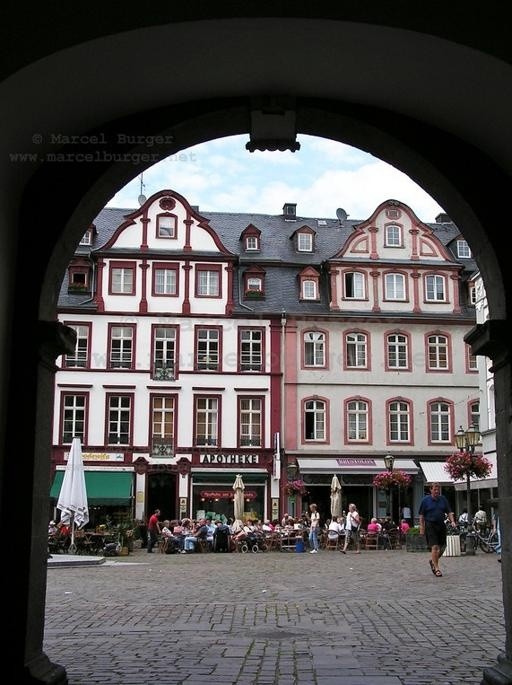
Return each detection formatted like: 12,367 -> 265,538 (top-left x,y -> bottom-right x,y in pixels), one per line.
455,421 -> 481,556
287,460 -> 299,518
384,450 -> 394,516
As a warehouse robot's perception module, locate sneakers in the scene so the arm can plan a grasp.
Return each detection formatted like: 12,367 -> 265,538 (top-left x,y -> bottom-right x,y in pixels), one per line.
181,550 -> 191,553
310,549 -> 318,553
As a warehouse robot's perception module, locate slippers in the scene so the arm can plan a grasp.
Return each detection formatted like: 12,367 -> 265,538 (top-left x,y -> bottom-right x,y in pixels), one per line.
340,550 -> 346,554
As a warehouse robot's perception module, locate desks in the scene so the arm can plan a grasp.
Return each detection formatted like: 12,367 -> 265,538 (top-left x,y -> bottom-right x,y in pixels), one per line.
92,532 -> 111,555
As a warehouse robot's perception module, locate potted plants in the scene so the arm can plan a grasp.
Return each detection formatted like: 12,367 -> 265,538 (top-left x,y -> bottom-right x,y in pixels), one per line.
442,524 -> 461,557
405,527 -> 430,552
245,289 -> 264,298
68,281 -> 87,293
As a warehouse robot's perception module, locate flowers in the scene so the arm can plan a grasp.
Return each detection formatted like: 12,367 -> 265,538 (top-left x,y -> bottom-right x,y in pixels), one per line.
443,451 -> 493,482
285,479 -> 311,497
373,470 -> 412,490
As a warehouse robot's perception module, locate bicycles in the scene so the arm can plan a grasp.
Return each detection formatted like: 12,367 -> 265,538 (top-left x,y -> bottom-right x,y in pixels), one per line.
459,517 -> 501,554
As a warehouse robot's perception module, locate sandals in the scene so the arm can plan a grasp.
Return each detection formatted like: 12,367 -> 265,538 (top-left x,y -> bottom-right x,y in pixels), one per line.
429,560 -> 442,577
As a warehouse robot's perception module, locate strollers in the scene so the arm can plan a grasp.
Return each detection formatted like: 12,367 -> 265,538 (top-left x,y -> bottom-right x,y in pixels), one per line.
239,524 -> 268,554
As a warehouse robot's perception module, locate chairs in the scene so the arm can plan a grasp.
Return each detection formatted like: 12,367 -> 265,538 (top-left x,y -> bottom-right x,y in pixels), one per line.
320,528 -> 401,550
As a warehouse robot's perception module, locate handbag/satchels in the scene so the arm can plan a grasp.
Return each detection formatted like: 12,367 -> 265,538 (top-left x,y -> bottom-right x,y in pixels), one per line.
351,525 -> 358,531
164,543 -> 179,554
319,519 -> 327,530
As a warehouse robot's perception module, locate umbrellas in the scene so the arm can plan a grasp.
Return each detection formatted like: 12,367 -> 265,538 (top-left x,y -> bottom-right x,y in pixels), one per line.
329,474 -> 342,518
56,436 -> 90,554
232,474 -> 246,522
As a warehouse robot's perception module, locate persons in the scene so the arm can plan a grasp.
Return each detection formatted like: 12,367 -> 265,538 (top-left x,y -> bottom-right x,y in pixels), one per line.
490,513 -> 501,563
305,503 -> 321,554
146,509 -> 162,554
456,508 -> 467,530
418,483 -> 456,577
339,504 -> 362,555
472,505 -> 487,536
160,512 -> 411,555
48,520 -> 71,540
